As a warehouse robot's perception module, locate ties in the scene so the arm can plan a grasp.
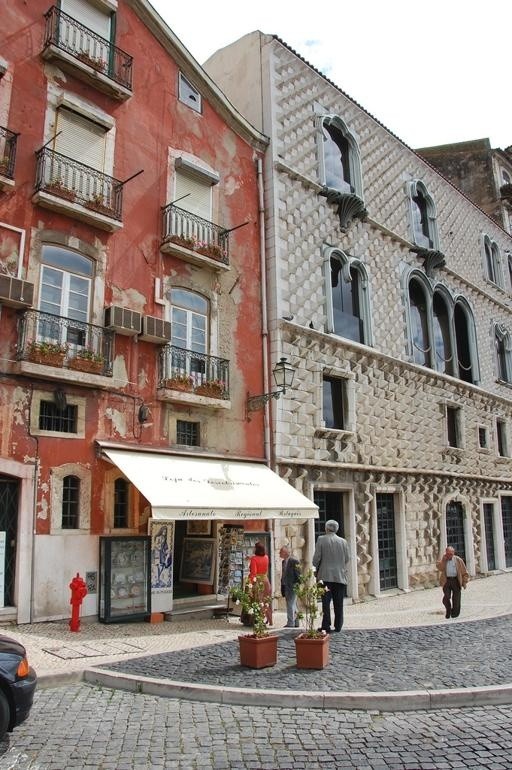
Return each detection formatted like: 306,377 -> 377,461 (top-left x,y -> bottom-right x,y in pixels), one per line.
282,560 -> 287,579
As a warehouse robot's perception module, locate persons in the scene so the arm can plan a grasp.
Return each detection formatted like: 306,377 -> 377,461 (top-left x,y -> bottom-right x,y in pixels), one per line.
438,547 -> 470,620
246,543 -> 273,626
312,520 -> 349,633
154,526 -> 167,584
279,545 -> 302,628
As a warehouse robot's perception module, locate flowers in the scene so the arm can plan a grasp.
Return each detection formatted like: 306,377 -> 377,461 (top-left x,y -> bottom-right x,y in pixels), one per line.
0,155 -> 228,263
25,338 -> 69,356
75,348 -> 106,362
169,374 -> 195,386
197,379 -> 224,394
232,573 -> 275,639
292,562 -> 332,640
78,47 -> 108,68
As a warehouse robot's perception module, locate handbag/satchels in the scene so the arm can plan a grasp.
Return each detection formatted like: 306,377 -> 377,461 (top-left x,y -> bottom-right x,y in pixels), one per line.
280,578 -> 286,597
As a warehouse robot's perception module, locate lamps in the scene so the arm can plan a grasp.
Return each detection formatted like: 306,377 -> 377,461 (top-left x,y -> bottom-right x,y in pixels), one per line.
127,395 -> 155,442
246,354 -> 296,421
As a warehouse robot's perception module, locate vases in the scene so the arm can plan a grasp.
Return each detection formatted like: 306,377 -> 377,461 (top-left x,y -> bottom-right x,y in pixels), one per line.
294,632 -> 331,670
28,349 -> 63,369
196,388 -> 221,399
237,634 -> 279,670
77,54 -> 103,72
71,357 -> 103,374
167,380 -> 192,392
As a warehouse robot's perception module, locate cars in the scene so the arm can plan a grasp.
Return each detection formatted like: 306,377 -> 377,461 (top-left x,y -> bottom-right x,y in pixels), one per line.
1,634 -> 37,745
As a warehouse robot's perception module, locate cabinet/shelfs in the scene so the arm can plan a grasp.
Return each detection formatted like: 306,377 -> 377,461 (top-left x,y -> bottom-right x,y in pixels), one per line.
98,534 -> 152,625
240,531 -> 273,597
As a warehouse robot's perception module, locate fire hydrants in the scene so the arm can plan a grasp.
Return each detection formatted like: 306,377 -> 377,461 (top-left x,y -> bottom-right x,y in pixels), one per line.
70,572 -> 88,633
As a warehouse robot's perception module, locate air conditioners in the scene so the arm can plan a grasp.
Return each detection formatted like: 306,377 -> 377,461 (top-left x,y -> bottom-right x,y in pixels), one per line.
138,314 -> 171,345
104,304 -> 142,340
0,273 -> 34,313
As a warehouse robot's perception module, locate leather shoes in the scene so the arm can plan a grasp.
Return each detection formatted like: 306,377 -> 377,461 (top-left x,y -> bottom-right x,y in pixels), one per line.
335,627 -> 342,632
317,625 -> 332,633
